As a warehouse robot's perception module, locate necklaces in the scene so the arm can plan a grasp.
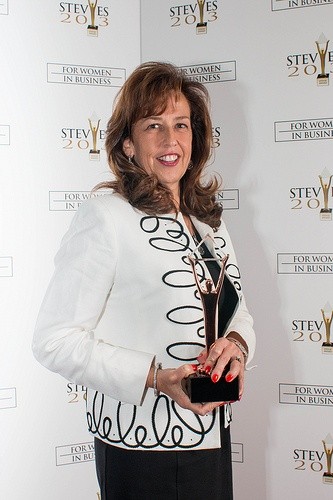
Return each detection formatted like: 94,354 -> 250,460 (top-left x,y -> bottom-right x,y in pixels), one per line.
190,233 -> 205,256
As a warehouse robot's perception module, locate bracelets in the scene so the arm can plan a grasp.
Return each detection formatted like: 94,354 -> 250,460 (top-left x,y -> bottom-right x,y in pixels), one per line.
153,362 -> 163,397
226,332 -> 248,359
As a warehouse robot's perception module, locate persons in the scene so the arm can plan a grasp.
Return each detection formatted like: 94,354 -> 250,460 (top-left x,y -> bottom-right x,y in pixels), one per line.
33,61 -> 257,500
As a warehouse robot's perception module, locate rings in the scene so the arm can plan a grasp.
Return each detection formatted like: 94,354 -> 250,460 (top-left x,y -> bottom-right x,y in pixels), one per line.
231,356 -> 242,364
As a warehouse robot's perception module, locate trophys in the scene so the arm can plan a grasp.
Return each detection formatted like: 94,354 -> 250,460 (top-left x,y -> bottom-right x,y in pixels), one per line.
181,233 -> 240,404
